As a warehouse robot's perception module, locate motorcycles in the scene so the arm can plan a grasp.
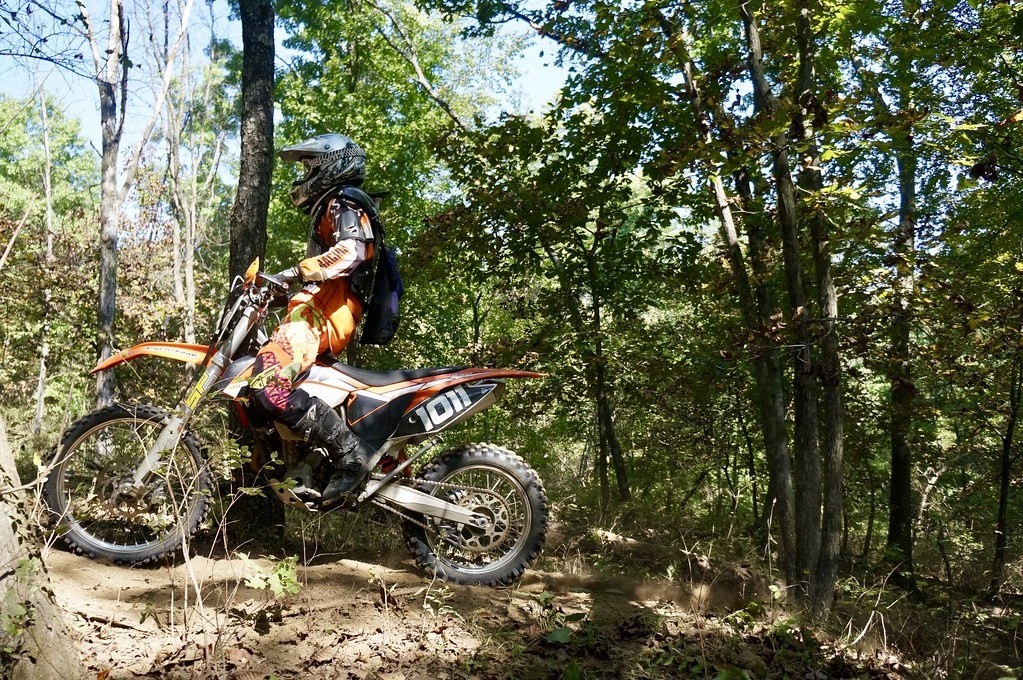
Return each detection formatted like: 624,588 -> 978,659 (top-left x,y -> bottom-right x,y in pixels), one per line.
48,257 -> 549,588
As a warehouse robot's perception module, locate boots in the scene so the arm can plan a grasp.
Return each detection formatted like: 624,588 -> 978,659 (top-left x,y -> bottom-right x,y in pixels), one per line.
289,396 -> 382,506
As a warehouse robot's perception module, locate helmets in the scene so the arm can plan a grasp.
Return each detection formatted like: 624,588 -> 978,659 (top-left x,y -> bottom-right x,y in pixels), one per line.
278,134 -> 367,217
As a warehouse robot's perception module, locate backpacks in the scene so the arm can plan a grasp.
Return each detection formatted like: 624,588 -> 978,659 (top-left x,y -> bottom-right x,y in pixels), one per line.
329,187 -> 404,345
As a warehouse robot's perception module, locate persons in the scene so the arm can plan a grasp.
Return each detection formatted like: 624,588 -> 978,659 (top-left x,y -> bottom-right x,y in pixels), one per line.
215,133 -> 382,537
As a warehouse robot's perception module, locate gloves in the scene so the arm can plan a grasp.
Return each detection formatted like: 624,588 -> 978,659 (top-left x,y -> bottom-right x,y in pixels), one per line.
267,265 -> 301,307
